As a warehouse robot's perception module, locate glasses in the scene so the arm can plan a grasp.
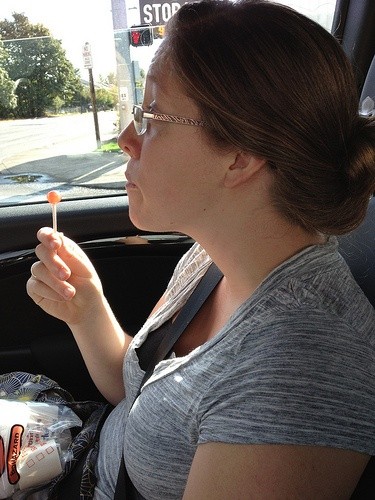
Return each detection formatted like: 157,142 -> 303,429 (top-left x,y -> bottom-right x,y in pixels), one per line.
131,102 -> 206,136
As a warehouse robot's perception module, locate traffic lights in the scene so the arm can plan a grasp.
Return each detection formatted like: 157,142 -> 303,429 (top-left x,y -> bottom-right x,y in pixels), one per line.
130,24 -> 153,46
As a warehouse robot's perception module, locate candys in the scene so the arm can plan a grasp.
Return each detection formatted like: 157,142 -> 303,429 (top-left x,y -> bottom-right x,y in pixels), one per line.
46,191 -> 60,204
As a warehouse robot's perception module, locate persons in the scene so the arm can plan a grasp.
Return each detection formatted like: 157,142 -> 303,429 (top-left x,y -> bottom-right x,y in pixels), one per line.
27,0 -> 375,500
